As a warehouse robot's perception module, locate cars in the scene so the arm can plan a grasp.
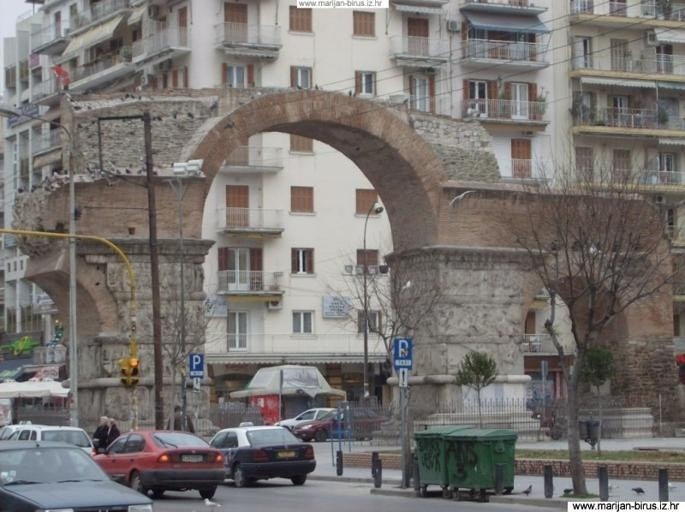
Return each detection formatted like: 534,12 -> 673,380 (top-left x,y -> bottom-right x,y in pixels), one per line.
274,407 -> 389,443
209,421 -> 317,488
0,420 -> 97,457
91,429 -> 226,500
0,439 -> 154,512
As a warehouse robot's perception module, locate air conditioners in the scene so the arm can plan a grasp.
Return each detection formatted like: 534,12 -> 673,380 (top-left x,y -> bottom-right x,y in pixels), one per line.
645,29 -> 659,48
377,264 -> 390,276
266,298 -> 281,311
446,19 -> 462,32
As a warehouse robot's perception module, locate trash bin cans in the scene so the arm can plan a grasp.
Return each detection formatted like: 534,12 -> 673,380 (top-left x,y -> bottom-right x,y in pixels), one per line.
413,425 -> 517,501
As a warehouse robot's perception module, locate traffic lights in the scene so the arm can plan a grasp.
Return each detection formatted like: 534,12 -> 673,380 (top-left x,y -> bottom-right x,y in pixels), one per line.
118,358 -> 140,388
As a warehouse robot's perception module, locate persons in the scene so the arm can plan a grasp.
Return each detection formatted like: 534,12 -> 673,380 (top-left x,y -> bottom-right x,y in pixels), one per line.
167,405 -> 195,434
51,64 -> 71,94
92,415 -> 110,442
100,418 -> 120,448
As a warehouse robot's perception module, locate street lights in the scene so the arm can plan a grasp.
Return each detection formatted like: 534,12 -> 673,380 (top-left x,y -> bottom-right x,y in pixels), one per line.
1,105 -> 82,429
